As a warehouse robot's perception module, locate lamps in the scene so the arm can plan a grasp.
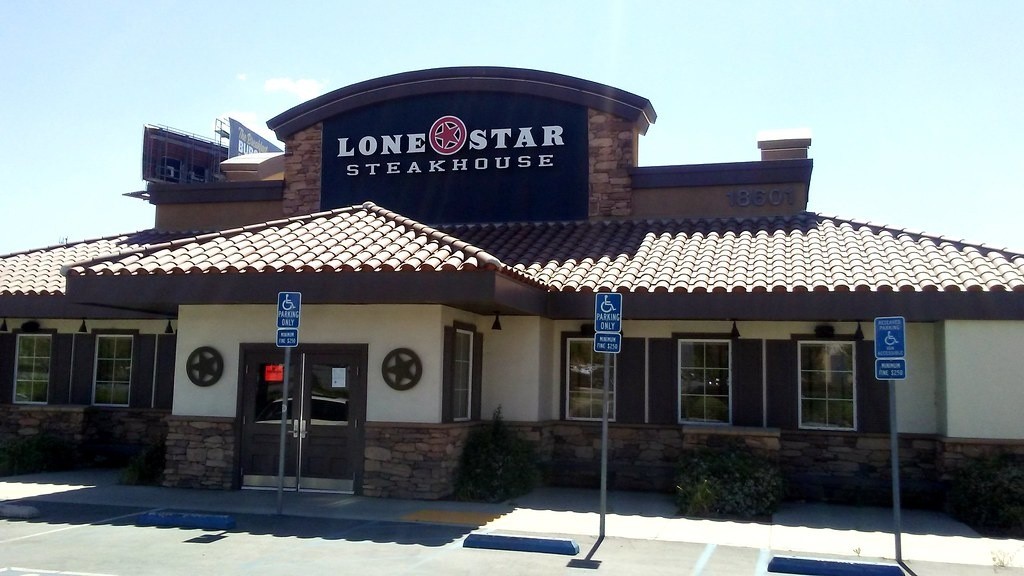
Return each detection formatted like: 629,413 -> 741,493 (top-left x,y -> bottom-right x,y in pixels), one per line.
730,321 -> 740,337
855,322 -> 864,340
0,317 -> 8,332
78,320 -> 87,333
164,320 -> 174,334
491,312 -> 502,331
